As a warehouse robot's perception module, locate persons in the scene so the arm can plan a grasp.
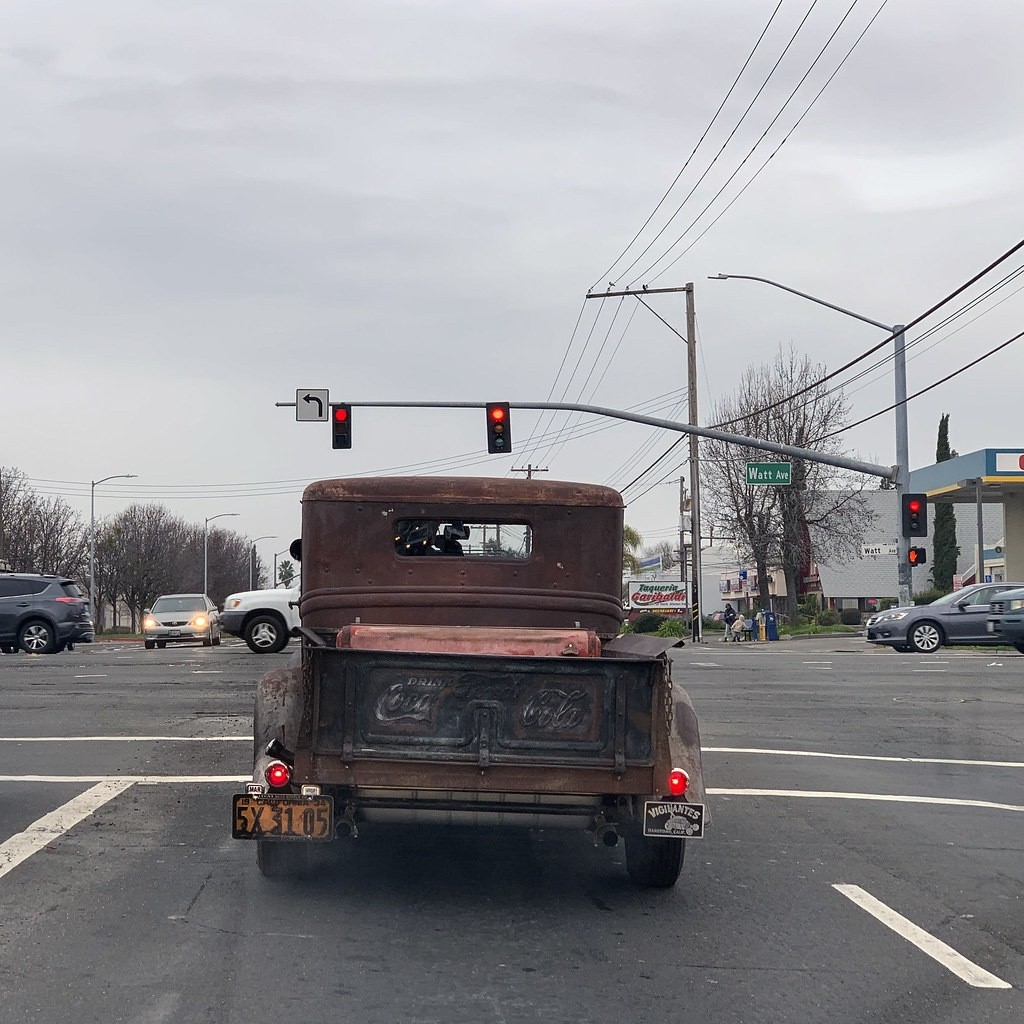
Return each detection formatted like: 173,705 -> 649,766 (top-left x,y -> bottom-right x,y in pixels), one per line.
722,603 -> 736,642
396,521 -> 445,557
731,615 -> 749,642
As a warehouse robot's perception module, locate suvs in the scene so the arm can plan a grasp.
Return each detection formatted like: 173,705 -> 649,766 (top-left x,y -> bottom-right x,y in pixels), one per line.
0,570 -> 96,654
218,577 -> 301,654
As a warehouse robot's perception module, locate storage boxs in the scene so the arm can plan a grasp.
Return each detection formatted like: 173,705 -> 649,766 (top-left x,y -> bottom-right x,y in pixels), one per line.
336,623 -> 601,659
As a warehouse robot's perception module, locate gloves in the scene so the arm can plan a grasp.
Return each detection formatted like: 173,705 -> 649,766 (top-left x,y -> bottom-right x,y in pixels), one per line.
730,614 -> 733,616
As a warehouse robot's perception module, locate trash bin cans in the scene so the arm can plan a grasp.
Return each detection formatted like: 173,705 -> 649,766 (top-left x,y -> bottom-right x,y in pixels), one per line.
765,612 -> 779,641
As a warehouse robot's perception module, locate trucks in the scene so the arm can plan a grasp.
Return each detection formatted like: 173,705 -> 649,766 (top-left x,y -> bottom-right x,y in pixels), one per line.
226,477 -> 706,881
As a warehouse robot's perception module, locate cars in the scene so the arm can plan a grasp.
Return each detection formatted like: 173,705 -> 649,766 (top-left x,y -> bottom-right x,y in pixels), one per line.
144,594 -> 222,648
864,582 -> 1024,653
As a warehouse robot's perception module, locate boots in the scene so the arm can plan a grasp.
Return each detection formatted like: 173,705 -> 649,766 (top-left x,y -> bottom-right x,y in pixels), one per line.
731,637 -> 736,642
723,637 -> 727,642
737,637 -> 742,642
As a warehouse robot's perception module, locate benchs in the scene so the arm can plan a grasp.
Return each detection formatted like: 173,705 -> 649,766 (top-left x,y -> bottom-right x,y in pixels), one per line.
730,629 -> 753,642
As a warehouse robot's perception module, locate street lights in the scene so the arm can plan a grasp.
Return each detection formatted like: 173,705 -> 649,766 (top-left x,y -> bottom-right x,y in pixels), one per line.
250,536 -> 278,591
89,475 -> 137,623
203,513 -> 241,593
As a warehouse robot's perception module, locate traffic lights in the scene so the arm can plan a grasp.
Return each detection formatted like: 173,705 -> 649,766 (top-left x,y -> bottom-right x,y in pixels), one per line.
486,402 -> 512,455
908,546 -> 926,567
332,404 -> 352,450
903,493 -> 928,538
